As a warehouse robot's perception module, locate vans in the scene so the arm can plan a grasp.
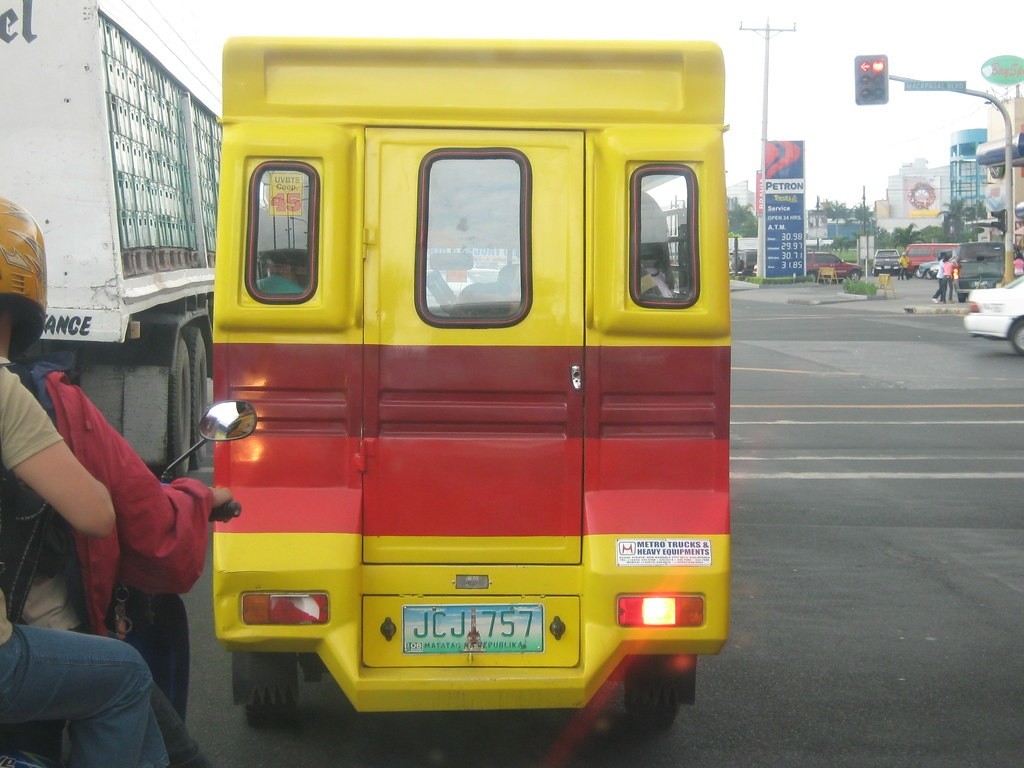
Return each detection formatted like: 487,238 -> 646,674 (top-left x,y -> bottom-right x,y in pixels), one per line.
903,243 -> 975,286
952,240 -> 1024,303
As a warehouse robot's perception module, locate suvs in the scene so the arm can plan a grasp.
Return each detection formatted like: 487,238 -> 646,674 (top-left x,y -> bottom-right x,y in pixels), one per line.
872,249 -> 905,279
805,251 -> 864,283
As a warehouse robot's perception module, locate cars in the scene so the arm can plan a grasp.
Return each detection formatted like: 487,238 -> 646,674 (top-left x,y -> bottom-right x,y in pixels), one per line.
918,255 -> 962,286
963,274 -> 1024,355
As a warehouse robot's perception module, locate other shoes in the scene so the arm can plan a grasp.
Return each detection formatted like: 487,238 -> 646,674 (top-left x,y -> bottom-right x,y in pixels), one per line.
932,298 -> 938,303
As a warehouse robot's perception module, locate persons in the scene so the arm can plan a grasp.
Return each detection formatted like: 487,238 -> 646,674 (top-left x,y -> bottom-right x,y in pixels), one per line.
942,257 -> 960,304
1013,253 -> 1024,278
0,195 -> 231,768
255,250 -> 307,294
899,252 -> 910,280
932,252 -> 946,304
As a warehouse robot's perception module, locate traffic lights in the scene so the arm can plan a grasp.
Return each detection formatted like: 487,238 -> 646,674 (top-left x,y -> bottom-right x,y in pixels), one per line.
991,209 -> 1007,233
854,55 -> 889,107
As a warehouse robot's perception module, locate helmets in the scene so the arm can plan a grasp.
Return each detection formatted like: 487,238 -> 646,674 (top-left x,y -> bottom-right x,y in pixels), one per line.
0,198 -> 47,357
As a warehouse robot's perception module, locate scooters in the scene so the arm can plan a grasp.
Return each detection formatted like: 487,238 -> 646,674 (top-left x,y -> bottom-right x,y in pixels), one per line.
2,402 -> 256,768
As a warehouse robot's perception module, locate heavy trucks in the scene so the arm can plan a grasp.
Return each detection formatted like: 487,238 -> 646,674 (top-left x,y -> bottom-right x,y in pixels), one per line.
0,0 -> 222,483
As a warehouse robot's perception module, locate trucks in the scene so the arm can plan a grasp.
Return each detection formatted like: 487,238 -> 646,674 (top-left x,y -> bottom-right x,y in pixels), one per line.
211,36 -> 732,732
